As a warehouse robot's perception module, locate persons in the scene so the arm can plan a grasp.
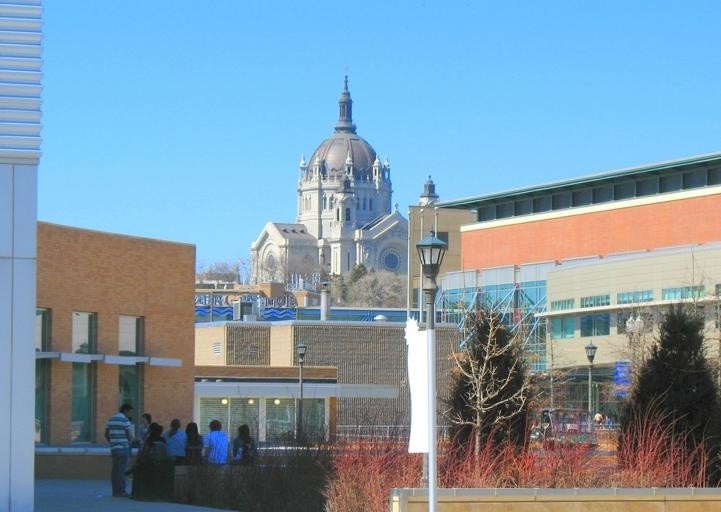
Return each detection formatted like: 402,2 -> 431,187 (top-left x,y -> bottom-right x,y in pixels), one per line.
594,411 -> 603,429
104,405 -> 259,498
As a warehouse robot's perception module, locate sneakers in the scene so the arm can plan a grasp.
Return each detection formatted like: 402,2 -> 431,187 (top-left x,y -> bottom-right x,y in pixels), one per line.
112,490 -> 128,497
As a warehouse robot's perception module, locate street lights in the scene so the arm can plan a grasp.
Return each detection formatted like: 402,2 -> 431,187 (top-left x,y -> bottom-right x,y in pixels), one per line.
626,315 -> 644,364
295,343 -> 307,432
415,228 -> 449,512
585,339 -> 598,436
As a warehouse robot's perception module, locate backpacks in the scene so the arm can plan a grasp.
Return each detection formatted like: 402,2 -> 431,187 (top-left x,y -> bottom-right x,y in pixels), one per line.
242,440 -> 256,461
